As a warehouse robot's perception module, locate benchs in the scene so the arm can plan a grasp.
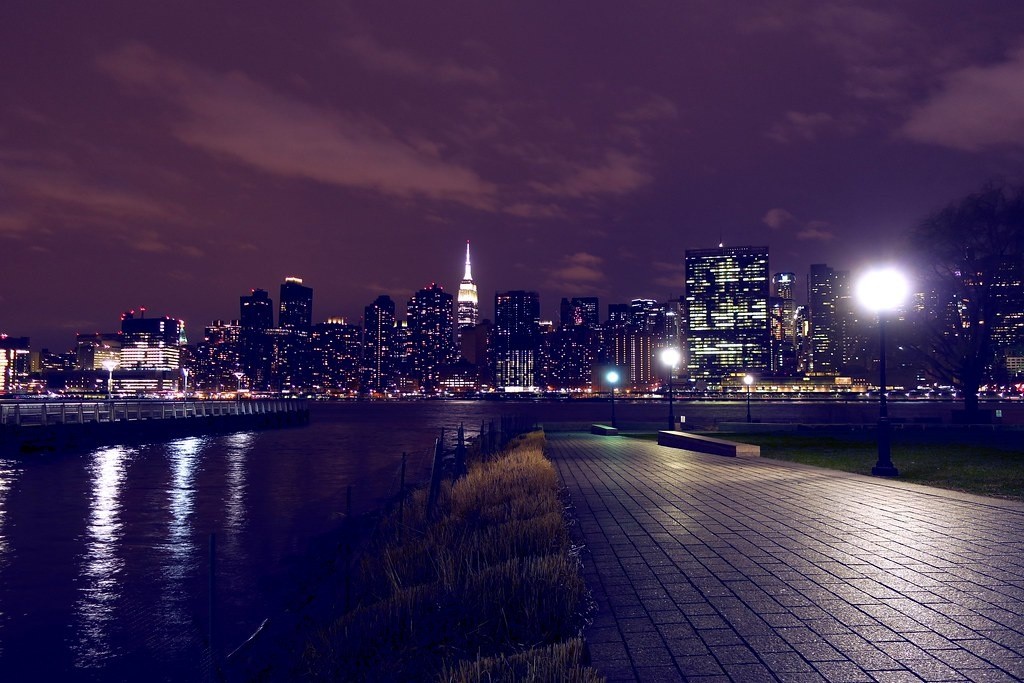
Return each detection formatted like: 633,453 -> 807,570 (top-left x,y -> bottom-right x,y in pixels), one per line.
658,430 -> 761,453
590,423 -> 617,437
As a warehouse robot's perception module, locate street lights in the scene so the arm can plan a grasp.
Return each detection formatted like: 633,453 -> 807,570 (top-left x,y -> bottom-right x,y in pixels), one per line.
858,264 -> 904,477
103,359 -> 119,399
607,372 -> 618,427
663,350 -> 678,430
744,376 -> 754,424
234,372 -> 246,399
